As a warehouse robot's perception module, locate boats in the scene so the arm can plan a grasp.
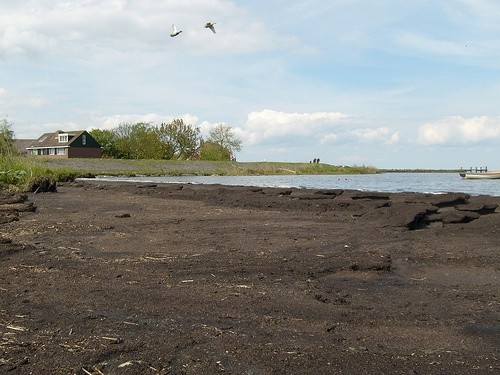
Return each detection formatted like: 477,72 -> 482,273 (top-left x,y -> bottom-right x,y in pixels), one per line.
459,170 -> 500,179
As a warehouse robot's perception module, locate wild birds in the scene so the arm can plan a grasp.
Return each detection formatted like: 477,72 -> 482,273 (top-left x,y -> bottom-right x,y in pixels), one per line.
204,21 -> 217,33
170,23 -> 182,37
